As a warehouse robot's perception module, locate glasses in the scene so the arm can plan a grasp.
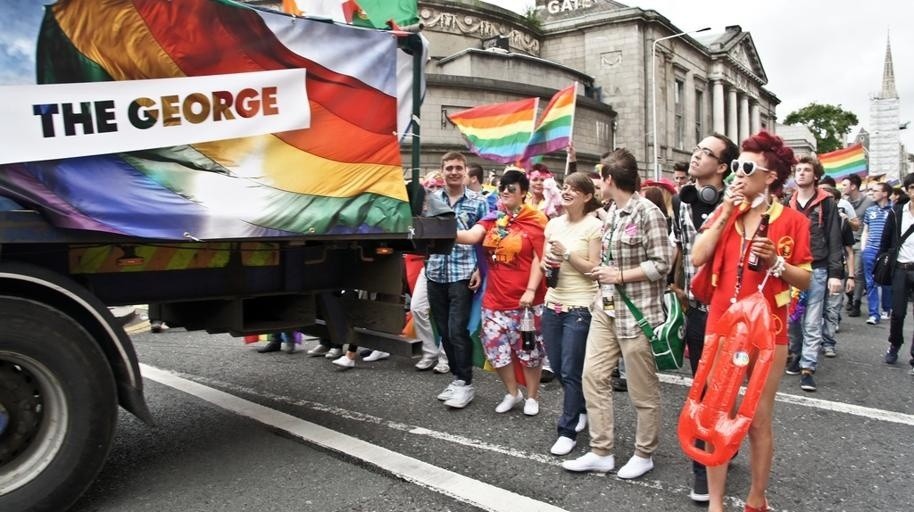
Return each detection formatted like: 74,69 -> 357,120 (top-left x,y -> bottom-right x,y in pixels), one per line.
693,145 -> 722,163
730,160 -> 769,176
498,185 -> 521,193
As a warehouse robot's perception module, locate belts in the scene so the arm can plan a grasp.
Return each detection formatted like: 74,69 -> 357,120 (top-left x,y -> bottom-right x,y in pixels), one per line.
544,302 -> 588,313
896,262 -> 914,270
689,301 -> 708,312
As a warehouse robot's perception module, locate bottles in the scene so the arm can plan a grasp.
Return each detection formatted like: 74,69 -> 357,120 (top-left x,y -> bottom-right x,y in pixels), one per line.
599,281 -> 615,310
544,253 -> 562,288
748,213 -> 769,272
520,306 -> 536,349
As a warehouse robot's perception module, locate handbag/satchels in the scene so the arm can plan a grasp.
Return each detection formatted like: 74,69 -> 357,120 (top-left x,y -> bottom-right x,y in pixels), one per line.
647,289 -> 687,372
870,246 -> 893,287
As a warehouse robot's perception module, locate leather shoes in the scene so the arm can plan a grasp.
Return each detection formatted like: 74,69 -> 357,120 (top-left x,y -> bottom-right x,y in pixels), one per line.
541,370 -> 555,382
613,377 -> 627,391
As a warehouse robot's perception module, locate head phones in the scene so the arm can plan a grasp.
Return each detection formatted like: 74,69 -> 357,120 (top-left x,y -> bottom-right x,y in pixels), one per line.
680,185 -> 724,205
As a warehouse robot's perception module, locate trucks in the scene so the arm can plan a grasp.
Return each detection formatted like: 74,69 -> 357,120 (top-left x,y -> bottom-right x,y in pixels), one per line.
0,1 -> 456,512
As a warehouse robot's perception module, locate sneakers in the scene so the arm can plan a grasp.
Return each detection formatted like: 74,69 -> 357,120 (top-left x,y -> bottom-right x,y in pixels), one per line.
846,301 -> 891,324
523,398 -> 539,416
690,472 -> 710,502
785,343 -> 835,391
550,413 -> 655,479
495,389 -> 523,414
415,357 -> 473,409
286,343 -> 294,353
307,344 -> 391,368
258,341 -> 281,353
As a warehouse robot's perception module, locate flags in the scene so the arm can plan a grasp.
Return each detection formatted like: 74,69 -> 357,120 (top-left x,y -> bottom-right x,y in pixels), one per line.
448,97 -> 540,163
0,0 -> 413,241
341,0 -> 419,29
519,84 -> 577,174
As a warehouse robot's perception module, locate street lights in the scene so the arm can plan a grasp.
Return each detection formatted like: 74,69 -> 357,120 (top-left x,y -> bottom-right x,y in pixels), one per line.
645,25 -> 711,185
899,120 -> 911,132
